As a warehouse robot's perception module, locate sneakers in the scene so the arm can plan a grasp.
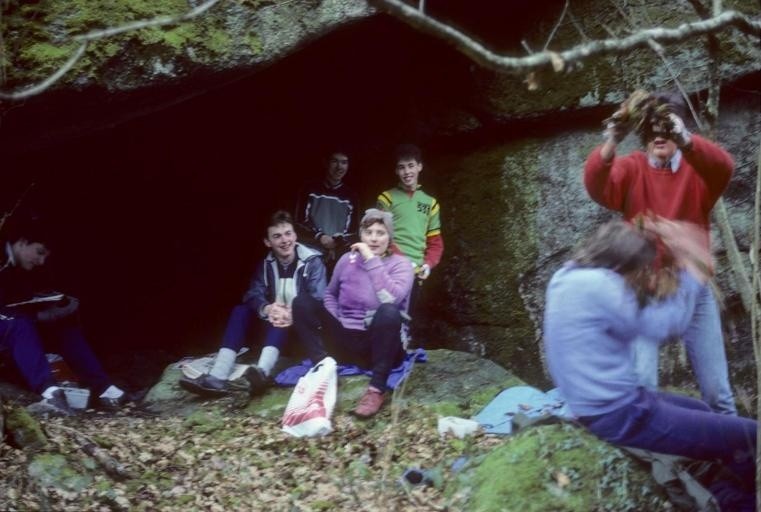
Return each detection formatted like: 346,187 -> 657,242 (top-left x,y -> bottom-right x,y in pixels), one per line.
179,372 -> 230,397
96,387 -> 149,416
32,387 -> 81,418
354,385 -> 386,419
243,363 -> 272,395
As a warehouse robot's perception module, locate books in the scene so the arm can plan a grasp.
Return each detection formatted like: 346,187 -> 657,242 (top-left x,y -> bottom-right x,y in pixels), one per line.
5,295 -> 63,307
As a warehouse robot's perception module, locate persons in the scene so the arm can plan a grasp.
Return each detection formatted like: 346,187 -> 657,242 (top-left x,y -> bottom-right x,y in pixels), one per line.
583,88 -> 738,415
545,215 -> 757,511
375,145 -> 445,350
291,209 -> 413,417
293,146 -> 359,274
178,210 -> 328,397
1,228 -> 148,413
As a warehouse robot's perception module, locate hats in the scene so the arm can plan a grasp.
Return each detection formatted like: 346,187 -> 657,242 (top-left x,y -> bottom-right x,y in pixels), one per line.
656,90 -> 688,117
